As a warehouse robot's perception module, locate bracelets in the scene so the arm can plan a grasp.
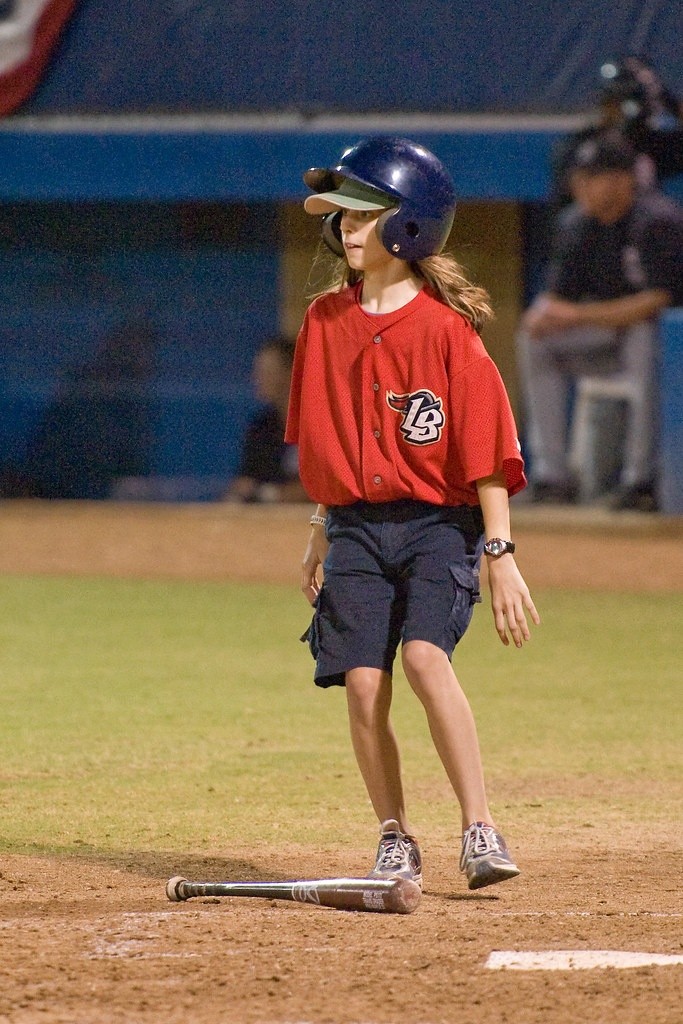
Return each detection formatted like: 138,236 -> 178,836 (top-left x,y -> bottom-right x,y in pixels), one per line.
309,515 -> 327,527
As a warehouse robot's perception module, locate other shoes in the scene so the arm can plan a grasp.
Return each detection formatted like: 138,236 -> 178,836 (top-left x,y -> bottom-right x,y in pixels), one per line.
612,483 -> 658,513
532,480 -> 576,504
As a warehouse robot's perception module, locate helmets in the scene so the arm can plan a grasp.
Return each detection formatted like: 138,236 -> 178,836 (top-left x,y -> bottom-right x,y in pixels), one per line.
303,135 -> 456,260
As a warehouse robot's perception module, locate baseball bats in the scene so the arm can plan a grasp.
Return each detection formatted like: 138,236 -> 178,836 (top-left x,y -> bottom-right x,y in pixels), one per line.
165,875 -> 423,915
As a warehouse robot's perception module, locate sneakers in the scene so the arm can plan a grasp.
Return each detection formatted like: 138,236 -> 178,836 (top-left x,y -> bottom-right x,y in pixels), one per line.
365,819 -> 422,887
458,819 -> 521,889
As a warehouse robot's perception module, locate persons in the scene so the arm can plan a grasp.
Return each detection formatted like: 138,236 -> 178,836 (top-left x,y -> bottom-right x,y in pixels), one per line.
228,338 -> 311,504
517,137 -> 683,519
281,133 -> 540,887
561,55 -> 670,200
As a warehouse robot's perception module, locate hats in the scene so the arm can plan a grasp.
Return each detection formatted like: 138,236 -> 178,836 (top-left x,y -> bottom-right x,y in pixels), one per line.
566,141 -> 633,174
304,178 -> 398,214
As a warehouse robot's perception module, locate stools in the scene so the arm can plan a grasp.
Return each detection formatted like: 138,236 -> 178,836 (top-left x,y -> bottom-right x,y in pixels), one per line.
564,374 -> 633,499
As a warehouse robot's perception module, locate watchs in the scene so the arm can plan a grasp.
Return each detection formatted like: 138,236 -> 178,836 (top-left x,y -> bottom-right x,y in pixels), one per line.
483,538 -> 516,557
